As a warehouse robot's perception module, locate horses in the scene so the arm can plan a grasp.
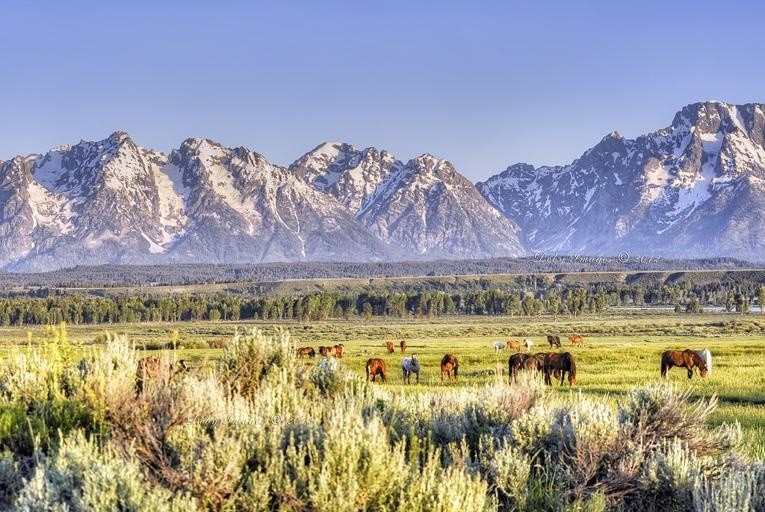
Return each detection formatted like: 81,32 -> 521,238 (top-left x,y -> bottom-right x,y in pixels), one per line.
568,335 -> 584,348
508,352 -> 576,388
440,352 -> 459,386
547,335 -> 560,348
660,349 -> 712,380
523,339 -> 534,351
507,340 -> 520,353
386,341 -> 394,353
295,343 -> 345,359
400,341 -> 407,352
365,358 -> 387,383
494,341 -> 507,353
401,353 -> 420,385
